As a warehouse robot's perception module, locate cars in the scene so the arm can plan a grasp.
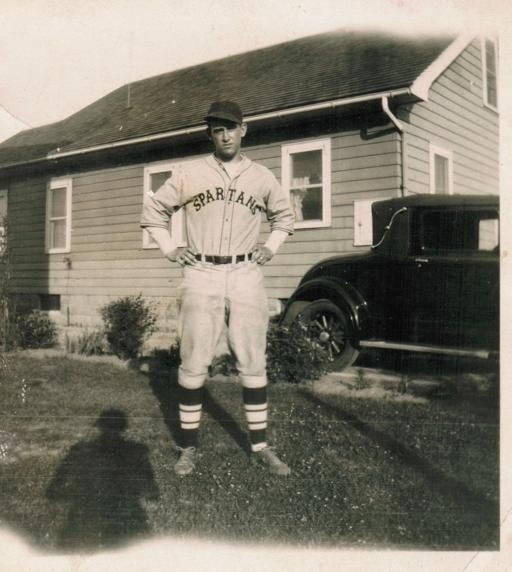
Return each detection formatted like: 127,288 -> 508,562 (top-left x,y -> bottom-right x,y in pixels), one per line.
279,194 -> 499,372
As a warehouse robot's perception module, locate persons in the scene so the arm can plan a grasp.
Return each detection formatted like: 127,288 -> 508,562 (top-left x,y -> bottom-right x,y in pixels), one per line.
141,104 -> 297,476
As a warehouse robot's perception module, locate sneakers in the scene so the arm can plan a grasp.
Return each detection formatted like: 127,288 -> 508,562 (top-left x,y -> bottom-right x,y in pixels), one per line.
174,446 -> 201,475
249,445 -> 292,477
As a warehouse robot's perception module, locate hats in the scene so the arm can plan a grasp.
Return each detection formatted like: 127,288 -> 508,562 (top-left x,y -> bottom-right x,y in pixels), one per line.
203,100 -> 244,125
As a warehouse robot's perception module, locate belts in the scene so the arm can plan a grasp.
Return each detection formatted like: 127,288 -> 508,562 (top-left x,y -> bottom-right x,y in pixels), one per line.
194,252 -> 253,265
